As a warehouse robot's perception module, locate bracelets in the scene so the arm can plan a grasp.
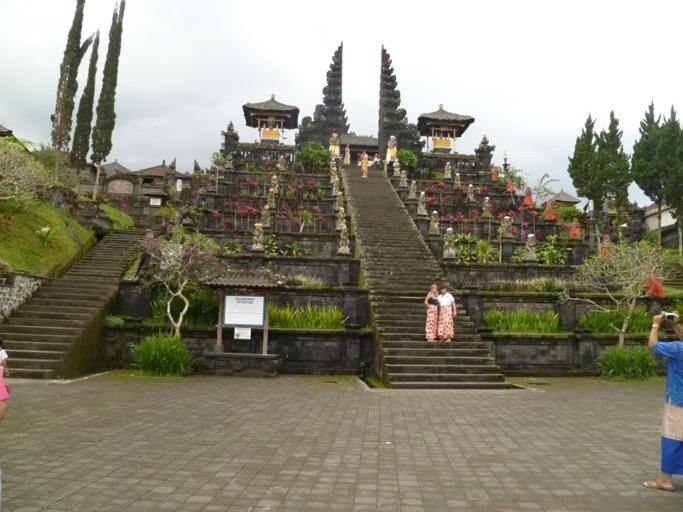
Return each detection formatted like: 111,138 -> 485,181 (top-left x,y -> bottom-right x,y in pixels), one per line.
651,324 -> 659,328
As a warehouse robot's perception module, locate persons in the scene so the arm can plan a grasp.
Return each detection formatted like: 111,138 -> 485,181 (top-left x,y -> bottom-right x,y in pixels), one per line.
223,153 -> 233,169
444,162 -> 493,219
251,221 -> 263,252
360,150 -> 368,179
428,210 -> 439,236
493,169 -> 582,238
269,175 -> 279,191
276,155 -> 285,171
598,235 -> 613,255
435,286 -> 456,342
328,132 -> 340,156
422,283 -> 439,343
0,339 -> 9,424
442,227 -> 456,259
434,132 -> 450,147
259,206 -> 270,227
261,123 -> 281,139
524,233 -> 536,262
342,144 -> 351,166
391,159 -> 427,216
264,189 -> 276,209
338,227 -> 351,255
329,157 -> 344,212
387,136 -> 397,163
641,309 -> 682,491
335,208 -> 347,231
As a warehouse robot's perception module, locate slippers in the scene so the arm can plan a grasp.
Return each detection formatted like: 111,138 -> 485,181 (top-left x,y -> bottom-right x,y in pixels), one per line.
642,479 -> 676,491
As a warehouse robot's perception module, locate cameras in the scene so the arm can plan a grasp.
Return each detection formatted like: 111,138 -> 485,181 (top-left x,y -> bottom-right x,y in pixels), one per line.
665,313 -> 676,320
